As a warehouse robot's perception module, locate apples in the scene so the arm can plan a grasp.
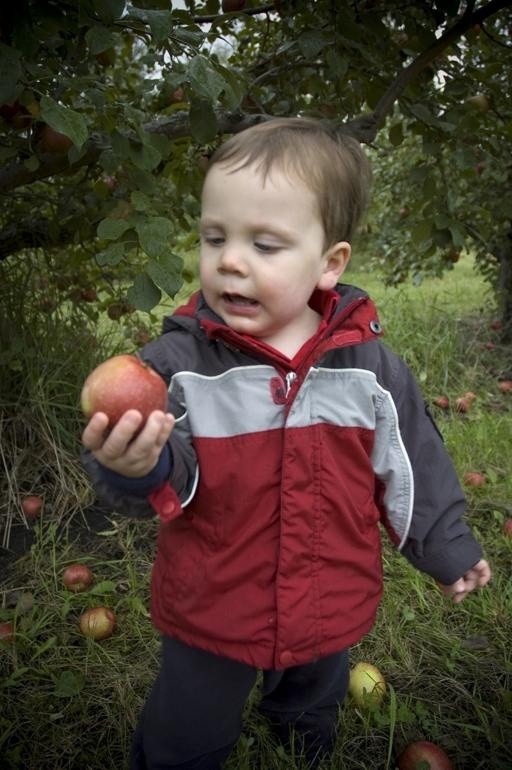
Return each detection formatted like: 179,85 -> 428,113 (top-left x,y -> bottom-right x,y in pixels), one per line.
464,472 -> 485,488
222,0 -> 512,265
349,661 -> 385,708
397,741 -> 451,770
76,605 -> 115,640
21,496 -> 44,520
0,71 -> 202,348
63,563 -> 94,592
433,309 -> 512,413
0,620 -> 16,642
77,354 -> 171,444
504,519 -> 512,538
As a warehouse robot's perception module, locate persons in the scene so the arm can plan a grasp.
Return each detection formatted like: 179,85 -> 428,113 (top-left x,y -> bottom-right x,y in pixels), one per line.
77,116 -> 491,770
